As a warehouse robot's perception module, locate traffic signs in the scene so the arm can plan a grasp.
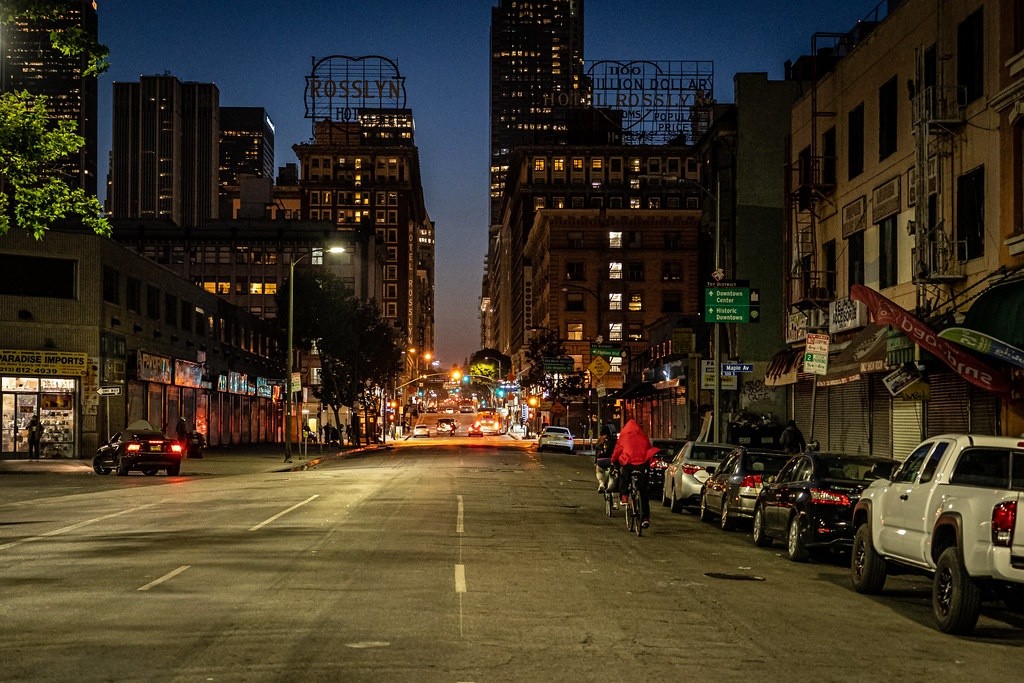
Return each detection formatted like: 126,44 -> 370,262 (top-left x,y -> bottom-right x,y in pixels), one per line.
96,385 -> 122,396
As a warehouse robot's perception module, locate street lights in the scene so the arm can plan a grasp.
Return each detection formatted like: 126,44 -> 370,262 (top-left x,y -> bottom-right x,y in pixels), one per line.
485,356 -> 502,382
286,245 -> 347,463
381,348 -> 415,444
416,354 -> 433,394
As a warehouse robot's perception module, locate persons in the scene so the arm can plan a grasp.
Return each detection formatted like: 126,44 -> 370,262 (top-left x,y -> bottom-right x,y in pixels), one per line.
324,422 -> 332,448
25,415 -> 44,460
611,419 -> 662,528
779,419 -> 807,454
346,425 -> 352,445
594,425 -> 618,493
176,417 -> 190,461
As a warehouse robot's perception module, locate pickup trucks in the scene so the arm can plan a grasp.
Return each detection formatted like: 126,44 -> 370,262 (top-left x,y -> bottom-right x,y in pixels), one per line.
848,433 -> 1024,635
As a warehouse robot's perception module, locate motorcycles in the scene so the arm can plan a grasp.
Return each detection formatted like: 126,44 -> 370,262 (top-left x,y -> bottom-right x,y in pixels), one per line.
189,433 -> 206,458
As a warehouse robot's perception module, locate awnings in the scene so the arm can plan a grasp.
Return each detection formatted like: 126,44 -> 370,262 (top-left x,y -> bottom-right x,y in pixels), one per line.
607,381 -> 660,399
961,278 -> 1024,349
816,321 -> 890,387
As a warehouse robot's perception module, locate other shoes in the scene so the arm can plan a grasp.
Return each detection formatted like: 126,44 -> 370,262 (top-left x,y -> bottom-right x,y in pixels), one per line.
598,484 -> 605,494
642,519 -> 649,528
620,495 -> 628,505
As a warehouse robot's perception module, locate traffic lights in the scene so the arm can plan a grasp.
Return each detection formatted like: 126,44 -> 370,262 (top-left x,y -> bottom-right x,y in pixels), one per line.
390,400 -> 397,408
527,398 -> 538,407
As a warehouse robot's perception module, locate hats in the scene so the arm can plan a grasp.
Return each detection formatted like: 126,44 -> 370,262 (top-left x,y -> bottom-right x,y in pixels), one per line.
600,426 -> 612,435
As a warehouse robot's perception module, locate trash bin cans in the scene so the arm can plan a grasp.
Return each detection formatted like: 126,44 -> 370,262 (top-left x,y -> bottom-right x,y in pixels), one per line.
730,427 -> 780,450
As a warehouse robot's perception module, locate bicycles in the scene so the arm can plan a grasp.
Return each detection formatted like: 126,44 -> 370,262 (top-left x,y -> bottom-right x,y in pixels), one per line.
611,459 -> 647,537
590,455 -> 618,518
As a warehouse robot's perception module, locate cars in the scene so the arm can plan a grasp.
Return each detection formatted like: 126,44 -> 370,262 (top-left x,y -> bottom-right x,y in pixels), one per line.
662,441 -> 736,513
413,398 -> 502,438
646,439 -> 689,498
751,451 -> 904,561
698,445 -> 797,530
92,429 -> 182,477
536,425 -> 576,452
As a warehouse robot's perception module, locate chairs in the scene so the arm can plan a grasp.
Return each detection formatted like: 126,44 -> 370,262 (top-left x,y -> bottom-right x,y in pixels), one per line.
830,470 -> 845,479
753,463 -> 764,471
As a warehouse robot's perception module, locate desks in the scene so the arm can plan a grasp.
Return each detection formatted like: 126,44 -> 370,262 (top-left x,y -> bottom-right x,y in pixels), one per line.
2,428 -> 26,434
